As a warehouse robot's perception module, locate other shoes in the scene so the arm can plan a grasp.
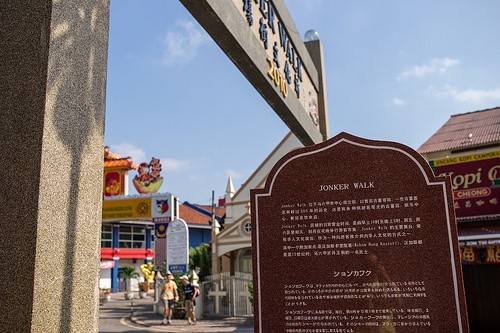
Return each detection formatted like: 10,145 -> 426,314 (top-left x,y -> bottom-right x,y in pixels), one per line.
192,321 -> 197,325
186,322 -> 191,325
167,320 -> 172,324
162,319 -> 166,324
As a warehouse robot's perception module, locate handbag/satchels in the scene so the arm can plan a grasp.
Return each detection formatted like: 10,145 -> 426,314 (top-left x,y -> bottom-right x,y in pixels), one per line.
191,284 -> 200,297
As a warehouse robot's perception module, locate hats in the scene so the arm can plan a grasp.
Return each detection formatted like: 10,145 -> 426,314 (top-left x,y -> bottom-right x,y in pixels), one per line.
166,271 -> 171,274
179,275 -> 188,280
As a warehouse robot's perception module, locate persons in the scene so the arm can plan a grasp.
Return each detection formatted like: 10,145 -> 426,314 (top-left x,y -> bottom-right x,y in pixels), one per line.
180,275 -> 197,326
160,274 -> 179,325
